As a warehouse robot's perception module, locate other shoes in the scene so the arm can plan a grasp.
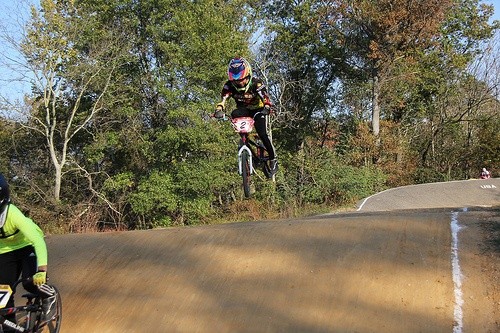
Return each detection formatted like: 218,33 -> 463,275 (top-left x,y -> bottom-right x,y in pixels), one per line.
270,160 -> 278,173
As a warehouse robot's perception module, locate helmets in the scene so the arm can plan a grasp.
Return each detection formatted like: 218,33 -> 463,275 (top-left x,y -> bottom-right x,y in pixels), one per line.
228,56 -> 252,96
0,174 -> 9,215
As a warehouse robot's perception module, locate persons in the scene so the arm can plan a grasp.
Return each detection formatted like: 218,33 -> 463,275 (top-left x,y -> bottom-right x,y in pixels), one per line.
482,168 -> 490,179
0,173 -> 56,333
215,57 -> 279,174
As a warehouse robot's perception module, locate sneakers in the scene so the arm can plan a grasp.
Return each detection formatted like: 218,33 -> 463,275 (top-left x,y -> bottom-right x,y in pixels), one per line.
40,297 -> 56,322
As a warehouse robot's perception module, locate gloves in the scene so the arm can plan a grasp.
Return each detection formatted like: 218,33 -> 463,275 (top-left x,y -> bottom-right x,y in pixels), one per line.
215,109 -> 223,118
32,270 -> 47,287
262,107 -> 270,115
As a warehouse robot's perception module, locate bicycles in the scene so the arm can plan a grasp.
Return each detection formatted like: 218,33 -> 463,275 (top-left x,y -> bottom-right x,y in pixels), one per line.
208,110 -> 279,198
0,276 -> 62,333
